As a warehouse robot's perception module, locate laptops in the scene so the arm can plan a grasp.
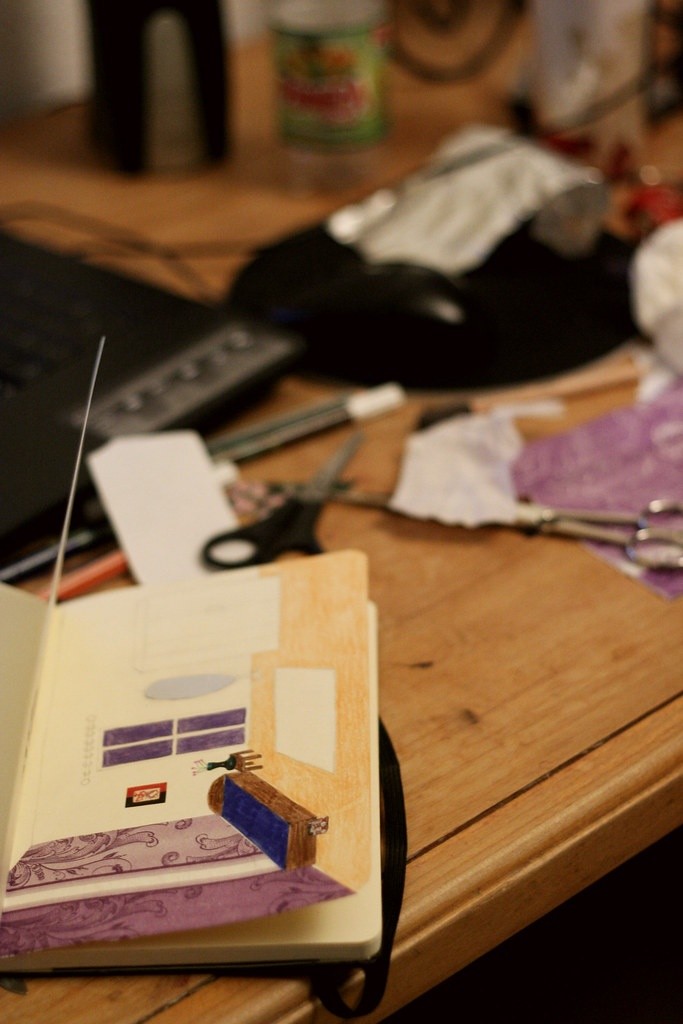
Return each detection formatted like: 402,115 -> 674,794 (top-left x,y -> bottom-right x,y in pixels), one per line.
0,229 -> 296,551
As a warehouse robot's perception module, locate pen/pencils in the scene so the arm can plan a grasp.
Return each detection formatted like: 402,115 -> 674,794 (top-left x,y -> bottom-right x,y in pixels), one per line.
0,520 -> 130,601
204,379 -> 407,465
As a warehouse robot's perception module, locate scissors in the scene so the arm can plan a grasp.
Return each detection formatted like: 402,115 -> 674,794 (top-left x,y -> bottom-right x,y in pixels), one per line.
202,431 -> 366,570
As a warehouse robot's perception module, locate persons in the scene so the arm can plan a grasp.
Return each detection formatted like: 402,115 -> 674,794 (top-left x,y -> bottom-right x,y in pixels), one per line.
82,1 -> 233,175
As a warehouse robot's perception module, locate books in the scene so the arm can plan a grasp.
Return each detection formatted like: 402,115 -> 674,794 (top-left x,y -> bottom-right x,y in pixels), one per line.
0,547 -> 377,969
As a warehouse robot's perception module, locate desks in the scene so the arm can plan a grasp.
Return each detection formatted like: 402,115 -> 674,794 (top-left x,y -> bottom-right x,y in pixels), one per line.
2,0 -> 682,1023
